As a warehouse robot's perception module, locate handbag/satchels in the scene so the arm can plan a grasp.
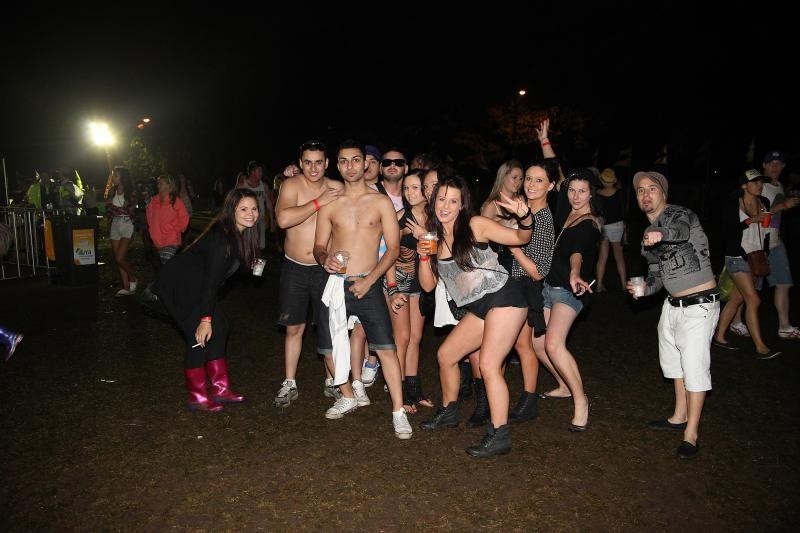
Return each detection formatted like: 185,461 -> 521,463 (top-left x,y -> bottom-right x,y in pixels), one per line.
746,249 -> 770,277
621,221 -> 639,247
448,298 -> 468,320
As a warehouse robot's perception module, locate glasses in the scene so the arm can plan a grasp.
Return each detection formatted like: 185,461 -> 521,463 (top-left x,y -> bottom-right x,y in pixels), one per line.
382,159 -> 406,167
300,141 -> 326,150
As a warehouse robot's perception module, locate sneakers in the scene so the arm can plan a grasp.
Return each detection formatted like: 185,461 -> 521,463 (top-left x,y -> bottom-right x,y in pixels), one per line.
272,355 -> 592,458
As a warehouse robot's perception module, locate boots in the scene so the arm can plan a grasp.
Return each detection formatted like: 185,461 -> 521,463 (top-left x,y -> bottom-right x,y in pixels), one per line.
0,327 -> 25,361
183,367 -> 224,413
205,358 -> 246,404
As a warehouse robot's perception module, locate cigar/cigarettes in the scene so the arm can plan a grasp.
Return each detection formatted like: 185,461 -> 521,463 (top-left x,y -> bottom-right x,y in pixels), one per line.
585,280 -> 595,290
191,343 -> 201,349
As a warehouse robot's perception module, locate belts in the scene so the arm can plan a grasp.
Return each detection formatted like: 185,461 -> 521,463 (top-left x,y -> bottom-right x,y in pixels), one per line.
668,294 -> 720,306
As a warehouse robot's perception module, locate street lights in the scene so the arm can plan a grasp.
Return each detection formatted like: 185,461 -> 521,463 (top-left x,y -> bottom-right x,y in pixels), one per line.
92,126 -> 112,170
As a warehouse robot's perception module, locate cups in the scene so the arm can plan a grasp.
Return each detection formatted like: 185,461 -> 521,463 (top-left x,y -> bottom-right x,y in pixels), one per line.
334,251 -> 349,274
419,232 -> 438,255
252,257 -> 267,276
760,212 -> 771,228
630,276 -> 645,297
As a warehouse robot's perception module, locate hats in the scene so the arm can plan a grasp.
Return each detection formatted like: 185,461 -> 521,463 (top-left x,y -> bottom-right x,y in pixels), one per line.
633,171 -> 668,196
764,150 -> 785,163
365,145 -> 381,160
738,169 -> 772,184
599,168 -> 617,184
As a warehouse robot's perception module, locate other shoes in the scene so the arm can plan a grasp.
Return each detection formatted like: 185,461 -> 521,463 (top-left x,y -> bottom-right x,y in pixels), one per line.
116,289 -> 136,296
622,282 -> 628,294
595,285 -> 607,294
677,441 -> 698,458
646,418 -> 688,432
130,278 -> 139,294
713,322 -> 800,360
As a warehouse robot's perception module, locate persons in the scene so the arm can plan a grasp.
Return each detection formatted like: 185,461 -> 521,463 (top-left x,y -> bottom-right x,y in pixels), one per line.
157,188 -> 266,411
414,174 -> 534,457
274,141 -> 345,404
590,168 -> 628,292
313,142 -> 413,441
106,167 -> 139,298
24,116 -> 800,457
628,171 -> 720,457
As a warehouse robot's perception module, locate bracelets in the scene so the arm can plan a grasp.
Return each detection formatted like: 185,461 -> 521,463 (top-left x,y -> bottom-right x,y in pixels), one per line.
197,314 -> 212,321
541,142 -> 550,146
387,282 -> 397,288
540,135 -> 548,141
387,287 -> 399,296
312,199 -> 320,211
783,202 -> 788,209
419,256 -> 429,261
517,207 -> 535,230
